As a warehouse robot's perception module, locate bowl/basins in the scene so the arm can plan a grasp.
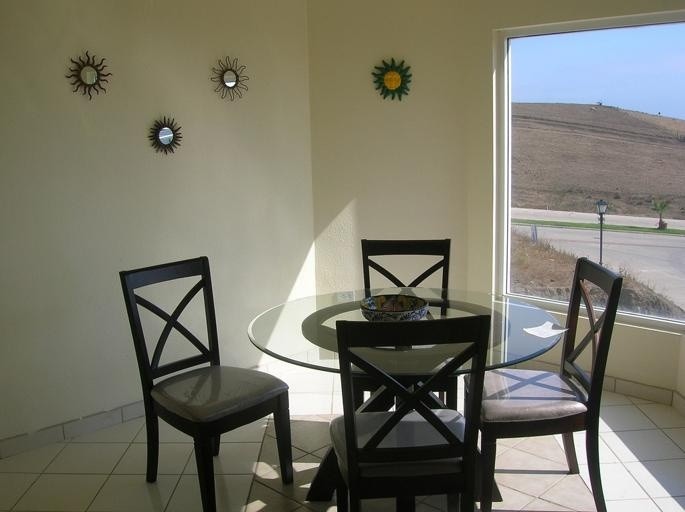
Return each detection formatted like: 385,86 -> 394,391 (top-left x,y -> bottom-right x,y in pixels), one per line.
359,295 -> 428,323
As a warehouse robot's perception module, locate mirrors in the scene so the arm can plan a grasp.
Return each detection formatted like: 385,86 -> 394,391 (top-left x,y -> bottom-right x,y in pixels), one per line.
146,115 -> 183,155
63,49 -> 113,102
210,54 -> 251,103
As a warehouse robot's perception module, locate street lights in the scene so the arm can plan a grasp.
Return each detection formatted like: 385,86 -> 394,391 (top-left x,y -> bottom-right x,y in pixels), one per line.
596,198 -> 608,267
